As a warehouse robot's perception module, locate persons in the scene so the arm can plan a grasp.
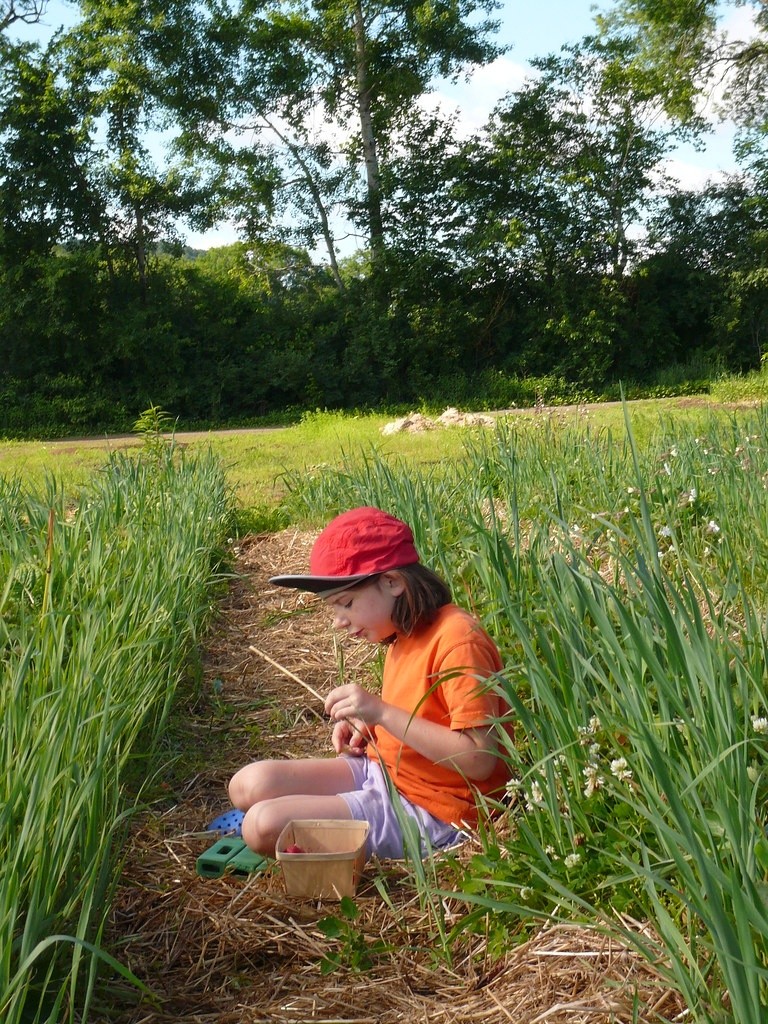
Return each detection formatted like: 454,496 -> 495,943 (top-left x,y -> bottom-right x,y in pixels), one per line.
205,506 -> 515,862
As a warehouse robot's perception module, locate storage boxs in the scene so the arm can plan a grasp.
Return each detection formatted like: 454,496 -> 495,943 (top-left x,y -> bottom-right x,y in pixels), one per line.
277,820 -> 371,901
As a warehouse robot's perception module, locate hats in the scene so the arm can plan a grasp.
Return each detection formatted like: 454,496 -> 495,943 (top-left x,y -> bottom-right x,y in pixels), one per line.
268,506 -> 420,600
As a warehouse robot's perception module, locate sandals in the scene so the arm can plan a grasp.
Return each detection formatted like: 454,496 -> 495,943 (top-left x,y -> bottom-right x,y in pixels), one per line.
207,806 -> 246,841
